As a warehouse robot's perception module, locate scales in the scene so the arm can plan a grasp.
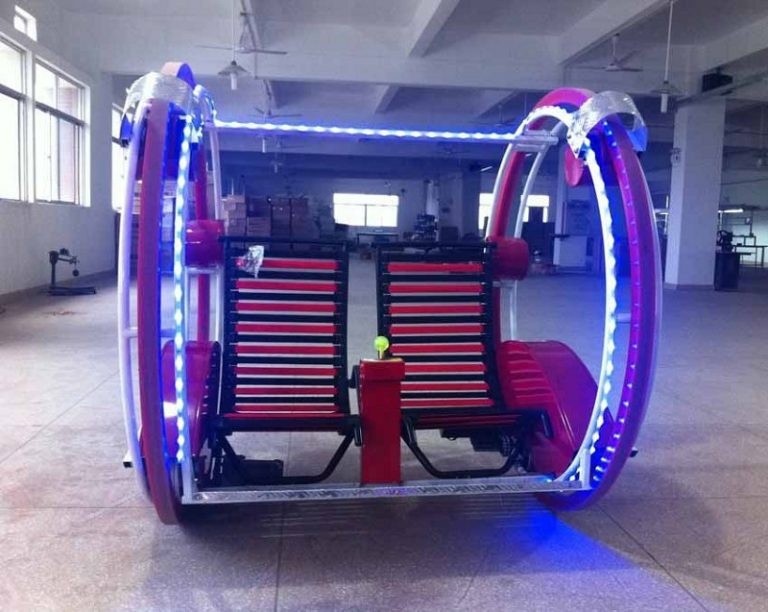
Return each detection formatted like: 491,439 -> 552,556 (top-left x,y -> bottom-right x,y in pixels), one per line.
47,248 -> 97,296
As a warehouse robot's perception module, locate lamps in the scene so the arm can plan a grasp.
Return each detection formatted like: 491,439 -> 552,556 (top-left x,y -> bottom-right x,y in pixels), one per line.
256,132 -> 272,153
650,0 -> 681,113
218,0 -> 248,90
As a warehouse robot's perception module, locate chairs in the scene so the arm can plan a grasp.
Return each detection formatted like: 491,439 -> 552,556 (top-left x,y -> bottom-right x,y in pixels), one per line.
216,233 -> 355,480
376,238 -> 552,475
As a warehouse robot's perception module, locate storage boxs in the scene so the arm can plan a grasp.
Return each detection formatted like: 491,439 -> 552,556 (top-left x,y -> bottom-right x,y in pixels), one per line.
116,180 -> 350,273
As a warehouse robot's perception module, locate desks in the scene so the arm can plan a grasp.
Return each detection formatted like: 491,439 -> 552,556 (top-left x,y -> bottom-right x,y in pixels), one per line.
357,232 -> 399,248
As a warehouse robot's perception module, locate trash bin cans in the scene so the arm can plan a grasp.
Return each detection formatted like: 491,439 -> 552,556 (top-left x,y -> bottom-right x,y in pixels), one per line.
716,253 -> 740,290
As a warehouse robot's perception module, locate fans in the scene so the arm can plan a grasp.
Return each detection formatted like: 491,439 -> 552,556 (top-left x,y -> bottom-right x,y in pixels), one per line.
249,100 -> 302,122
574,35 -> 643,72
481,104 -> 516,127
194,12 -> 288,55
